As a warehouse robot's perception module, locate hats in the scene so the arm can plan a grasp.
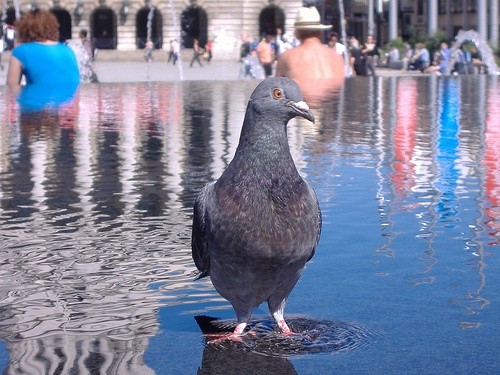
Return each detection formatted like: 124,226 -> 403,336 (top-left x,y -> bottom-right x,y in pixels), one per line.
285,5 -> 334,32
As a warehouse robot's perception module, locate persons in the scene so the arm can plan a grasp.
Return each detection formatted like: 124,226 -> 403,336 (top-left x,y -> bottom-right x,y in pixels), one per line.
239,5 -> 485,77
145,34 -> 216,67
6,8 -> 80,86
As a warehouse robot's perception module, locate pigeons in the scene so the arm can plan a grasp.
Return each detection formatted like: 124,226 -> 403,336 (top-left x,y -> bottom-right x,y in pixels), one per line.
191,75 -> 322,346
